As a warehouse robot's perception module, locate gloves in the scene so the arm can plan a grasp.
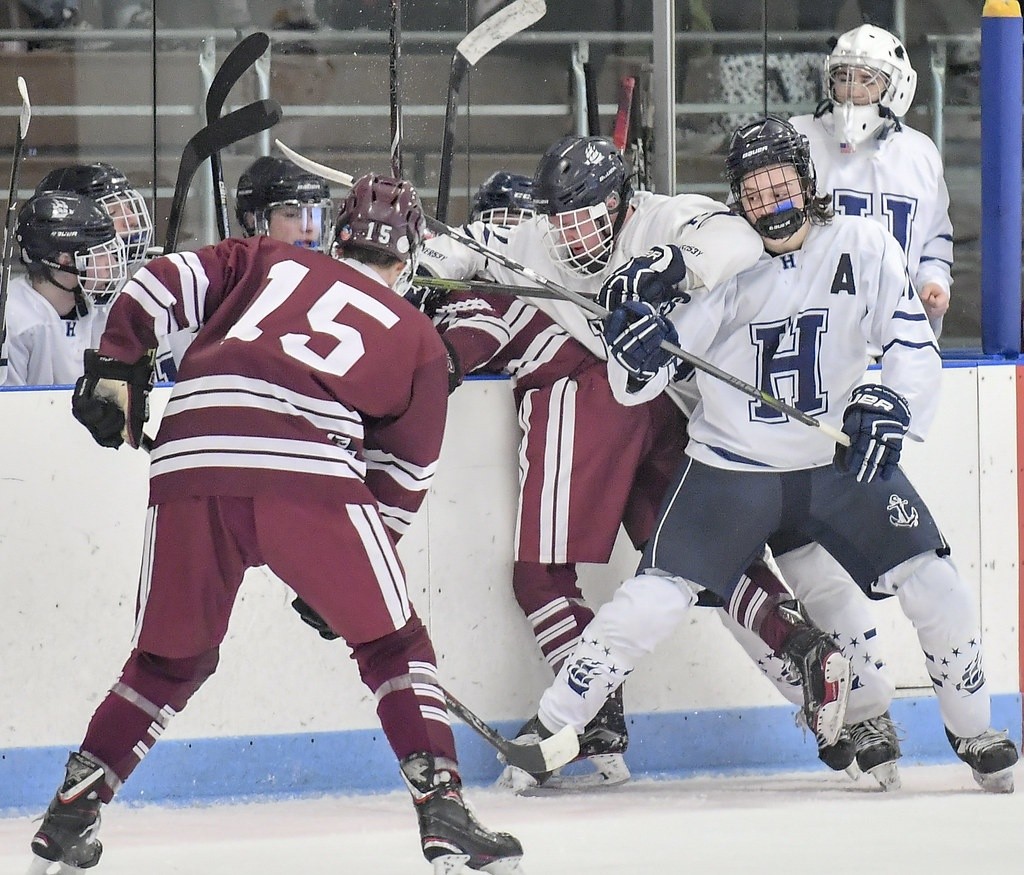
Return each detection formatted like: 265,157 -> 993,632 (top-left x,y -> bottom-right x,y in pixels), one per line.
595,245 -> 690,311
832,382 -> 912,486
603,299 -> 683,383
290,598 -> 341,641
71,349 -> 156,450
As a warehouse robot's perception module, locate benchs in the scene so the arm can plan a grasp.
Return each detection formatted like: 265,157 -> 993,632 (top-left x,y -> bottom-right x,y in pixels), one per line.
1,22 -> 982,199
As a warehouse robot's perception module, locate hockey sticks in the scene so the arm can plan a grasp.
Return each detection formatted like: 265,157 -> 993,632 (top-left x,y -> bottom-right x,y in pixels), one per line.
130,424 -> 587,776
385,0 -> 406,183
271,134 -> 856,451
156,27 -> 286,256
608,69 -> 655,195
0,72 -> 35,392
434,0 -> 550,227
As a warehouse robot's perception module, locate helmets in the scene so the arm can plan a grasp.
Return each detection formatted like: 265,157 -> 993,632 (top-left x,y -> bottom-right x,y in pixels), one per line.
724,113 -> 820,241
14,188 -> 129,307
529,134 -> 635,279
819,23 -> 918,146
33,162 -> 154,269
234,155 -> 335,252
330,173 -> 427,298
470,171 -> 538,225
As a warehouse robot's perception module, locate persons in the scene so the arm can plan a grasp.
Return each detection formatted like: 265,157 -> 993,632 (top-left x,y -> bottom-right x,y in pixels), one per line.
30,173 -> 526,875
782,23 -> 953,338
499,113 -> 1024,802
235,135 -> 901,794
0,162 -> 167,395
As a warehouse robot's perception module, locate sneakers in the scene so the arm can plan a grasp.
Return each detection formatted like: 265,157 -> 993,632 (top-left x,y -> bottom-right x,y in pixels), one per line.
793,707 -> 861,781
845,708 -> 904,792
944,725 -> 1020,794
544,692 -> 633,789
399,751 -> 523,874
776,599 -> 856,748
495,720 -> 559,789
30,750 -> 103,868
